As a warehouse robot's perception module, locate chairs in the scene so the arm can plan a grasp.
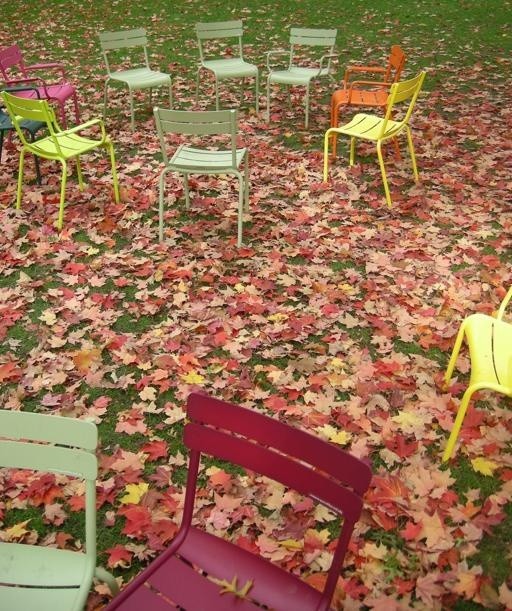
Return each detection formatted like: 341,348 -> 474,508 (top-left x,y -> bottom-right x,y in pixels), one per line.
323,70 -> 425,209
98,29 -> 173,130
266,27 -> 338,129
97,391 -> 372,610
152,105 -> 251,250
1,85 -> 53,185
193,22 -> 259,112
441,284 -> 512,470
2,43 -> 81,130
0,408 -> 119,610
1,91 -> 119,232
330,46 -> 406,162
